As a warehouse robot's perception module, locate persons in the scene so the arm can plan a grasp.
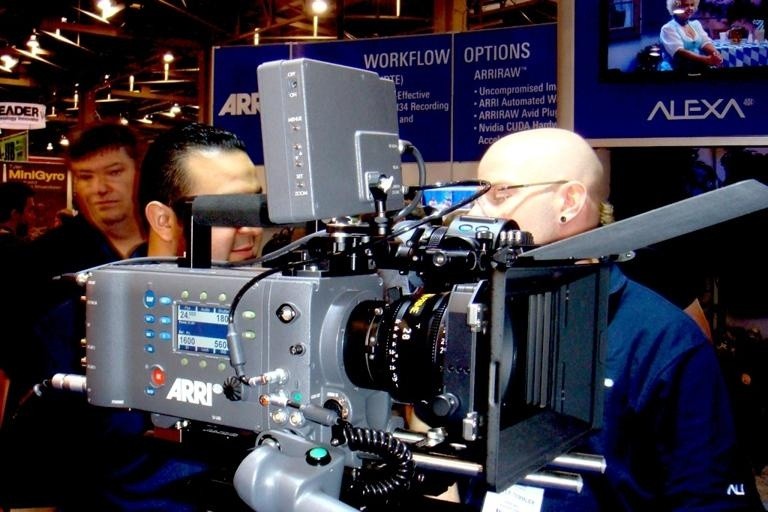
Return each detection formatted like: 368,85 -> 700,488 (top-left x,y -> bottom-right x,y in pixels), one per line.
0,178 -> 39,266
704,144 -> 767,345
602,142 -> 716,346
8,120 -> 271,512
0,114 -> 151,512
660,0 -> 725,70
410,127 -> 742,512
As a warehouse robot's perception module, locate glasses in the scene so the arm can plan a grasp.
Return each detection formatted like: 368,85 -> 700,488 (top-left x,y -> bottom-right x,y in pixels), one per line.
482,179 -> 564,206
